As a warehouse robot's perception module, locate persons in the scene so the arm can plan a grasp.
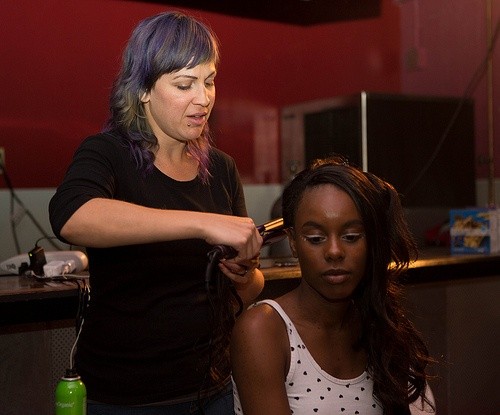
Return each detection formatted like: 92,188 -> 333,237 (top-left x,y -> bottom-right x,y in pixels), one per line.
49,12 -> 265,415
227,158 -> 438,415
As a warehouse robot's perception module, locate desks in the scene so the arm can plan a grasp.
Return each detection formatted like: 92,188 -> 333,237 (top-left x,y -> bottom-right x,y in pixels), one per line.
0,245 -> 500,415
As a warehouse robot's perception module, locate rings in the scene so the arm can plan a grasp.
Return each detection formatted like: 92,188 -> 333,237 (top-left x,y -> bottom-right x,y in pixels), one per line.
238,267 -> 248,276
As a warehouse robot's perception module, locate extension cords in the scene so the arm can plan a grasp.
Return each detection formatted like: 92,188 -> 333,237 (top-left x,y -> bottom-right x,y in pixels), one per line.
0,251 -> 88,277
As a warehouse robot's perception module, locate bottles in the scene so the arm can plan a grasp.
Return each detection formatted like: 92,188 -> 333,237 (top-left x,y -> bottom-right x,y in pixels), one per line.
54,367 -> 87,415
